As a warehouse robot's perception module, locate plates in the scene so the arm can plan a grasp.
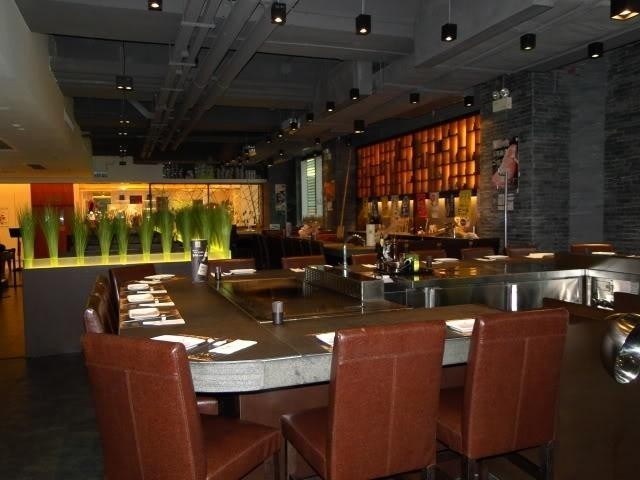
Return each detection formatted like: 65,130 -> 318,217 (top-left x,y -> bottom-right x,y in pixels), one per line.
126,275 -> 170,319
232,269 -> 256,274
434,252 -> 554,262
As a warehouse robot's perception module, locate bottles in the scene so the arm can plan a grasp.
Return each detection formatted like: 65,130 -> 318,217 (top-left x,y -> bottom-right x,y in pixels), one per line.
160,161 -> 256,179
191,240 -> 208,283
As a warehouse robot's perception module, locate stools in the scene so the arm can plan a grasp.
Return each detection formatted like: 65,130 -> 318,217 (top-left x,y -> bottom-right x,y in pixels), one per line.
2,248 -> 17,289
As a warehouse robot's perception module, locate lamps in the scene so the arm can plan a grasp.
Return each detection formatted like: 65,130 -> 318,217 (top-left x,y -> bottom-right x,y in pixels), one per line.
148,1 -> 163,12
116,40 -> 134,90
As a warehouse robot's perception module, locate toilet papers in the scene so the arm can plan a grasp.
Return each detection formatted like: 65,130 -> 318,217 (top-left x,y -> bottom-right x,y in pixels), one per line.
365,223 -> 377,247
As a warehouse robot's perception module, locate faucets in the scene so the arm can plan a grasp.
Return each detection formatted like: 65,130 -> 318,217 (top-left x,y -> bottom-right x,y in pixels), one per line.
342,233 -> 365,277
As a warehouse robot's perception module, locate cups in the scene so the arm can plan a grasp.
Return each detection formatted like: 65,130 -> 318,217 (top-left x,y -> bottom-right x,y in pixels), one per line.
272,300 -> 285,323
215,266 -> 222,280
